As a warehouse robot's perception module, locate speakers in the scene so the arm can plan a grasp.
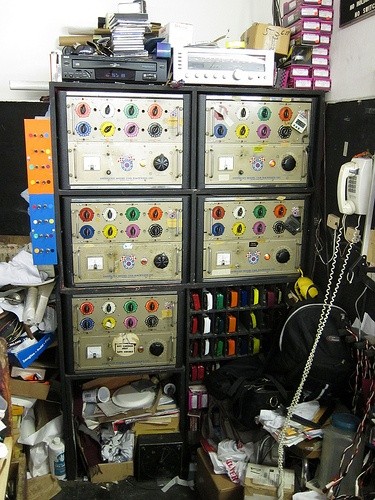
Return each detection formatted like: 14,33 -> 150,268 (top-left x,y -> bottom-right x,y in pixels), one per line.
172,46 -> 275,87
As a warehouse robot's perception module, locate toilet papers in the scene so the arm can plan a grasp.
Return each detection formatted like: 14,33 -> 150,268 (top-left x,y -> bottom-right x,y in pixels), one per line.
23,287 -> 38,325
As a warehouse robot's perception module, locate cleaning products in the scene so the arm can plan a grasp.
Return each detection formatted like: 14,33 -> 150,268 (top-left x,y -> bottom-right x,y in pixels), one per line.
48,437 -> 66,480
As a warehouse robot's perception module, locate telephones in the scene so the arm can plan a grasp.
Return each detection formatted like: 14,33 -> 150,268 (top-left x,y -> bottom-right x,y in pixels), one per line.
336,157 -> 375,215
